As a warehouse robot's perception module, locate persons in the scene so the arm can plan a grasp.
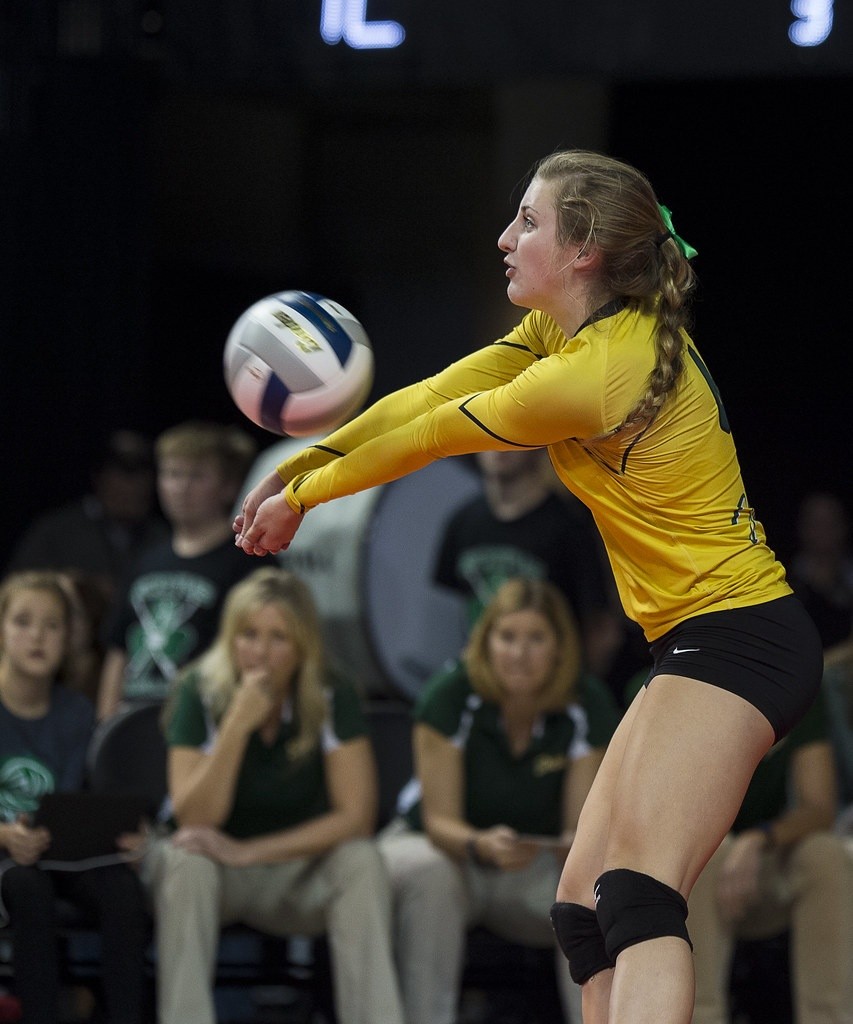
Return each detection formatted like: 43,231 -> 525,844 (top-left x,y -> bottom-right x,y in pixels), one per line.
233,146 -> 827,1024
1,423 -> 852,1024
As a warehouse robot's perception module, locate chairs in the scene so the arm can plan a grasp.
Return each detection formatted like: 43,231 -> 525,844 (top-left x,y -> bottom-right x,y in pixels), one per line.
86,705 -> 170,832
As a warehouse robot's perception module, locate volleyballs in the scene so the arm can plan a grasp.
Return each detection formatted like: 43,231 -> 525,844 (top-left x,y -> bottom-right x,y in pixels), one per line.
224,289 -> 377,439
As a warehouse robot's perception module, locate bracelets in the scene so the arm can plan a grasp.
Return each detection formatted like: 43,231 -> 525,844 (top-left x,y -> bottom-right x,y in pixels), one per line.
757,822 -> 777,851
461,827 -> 489,867
